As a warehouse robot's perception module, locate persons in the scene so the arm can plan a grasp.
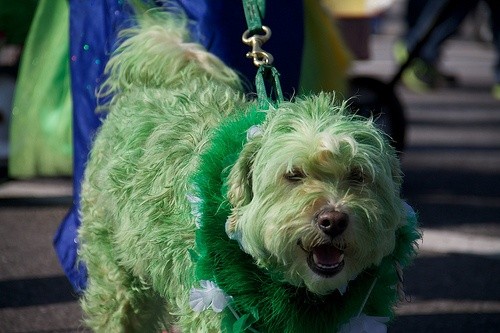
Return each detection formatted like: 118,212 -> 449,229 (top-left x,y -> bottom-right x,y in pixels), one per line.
53,0 -> 305,296
393,0 -> 499,101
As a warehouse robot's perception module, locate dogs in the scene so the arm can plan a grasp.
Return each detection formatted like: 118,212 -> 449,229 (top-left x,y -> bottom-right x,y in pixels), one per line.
74,1 -> 423,333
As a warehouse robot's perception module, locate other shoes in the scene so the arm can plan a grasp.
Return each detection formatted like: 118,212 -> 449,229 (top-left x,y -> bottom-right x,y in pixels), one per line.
393,39 -> 455,95
